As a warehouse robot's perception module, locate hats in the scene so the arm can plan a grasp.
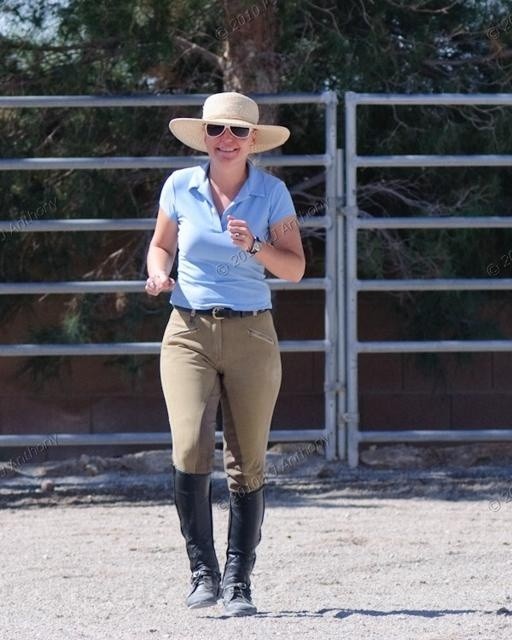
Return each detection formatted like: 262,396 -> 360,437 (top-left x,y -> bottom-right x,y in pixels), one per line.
166,90 -> 292,158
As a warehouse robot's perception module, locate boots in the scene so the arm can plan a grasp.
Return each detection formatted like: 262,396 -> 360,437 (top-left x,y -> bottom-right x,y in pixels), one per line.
220,485 -> 266,618
171,464 -> 221,611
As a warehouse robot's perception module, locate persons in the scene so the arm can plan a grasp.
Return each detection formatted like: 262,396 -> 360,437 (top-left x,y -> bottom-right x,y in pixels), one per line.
144,90 -> 306,617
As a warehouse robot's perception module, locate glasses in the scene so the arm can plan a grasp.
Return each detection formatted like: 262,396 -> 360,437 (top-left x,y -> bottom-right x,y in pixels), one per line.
202,120 -> 253,140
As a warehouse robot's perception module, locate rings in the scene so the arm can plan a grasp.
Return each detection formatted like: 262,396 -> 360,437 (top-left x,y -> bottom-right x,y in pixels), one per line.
149,281 -> 156,289
234,232 -> 240,240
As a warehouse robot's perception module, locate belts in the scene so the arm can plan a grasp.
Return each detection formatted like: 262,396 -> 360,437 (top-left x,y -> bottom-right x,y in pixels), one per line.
173,304 -> 270,320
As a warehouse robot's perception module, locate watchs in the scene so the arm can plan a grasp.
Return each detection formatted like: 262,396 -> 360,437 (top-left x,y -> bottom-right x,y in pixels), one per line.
247,234 -> 262,257
